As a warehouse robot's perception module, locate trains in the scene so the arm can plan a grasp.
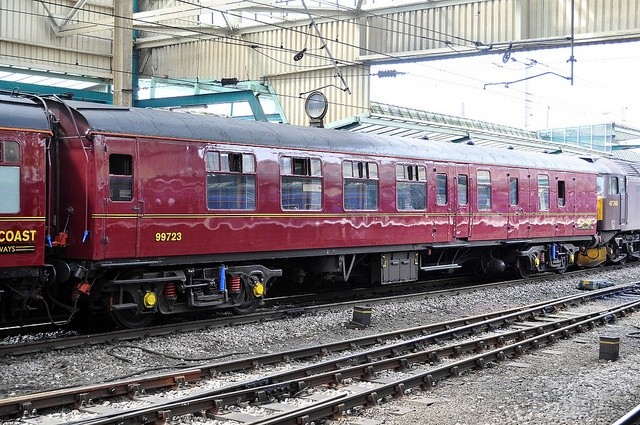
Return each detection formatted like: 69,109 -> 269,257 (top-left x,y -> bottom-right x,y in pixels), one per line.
0,88 -> 640,342
